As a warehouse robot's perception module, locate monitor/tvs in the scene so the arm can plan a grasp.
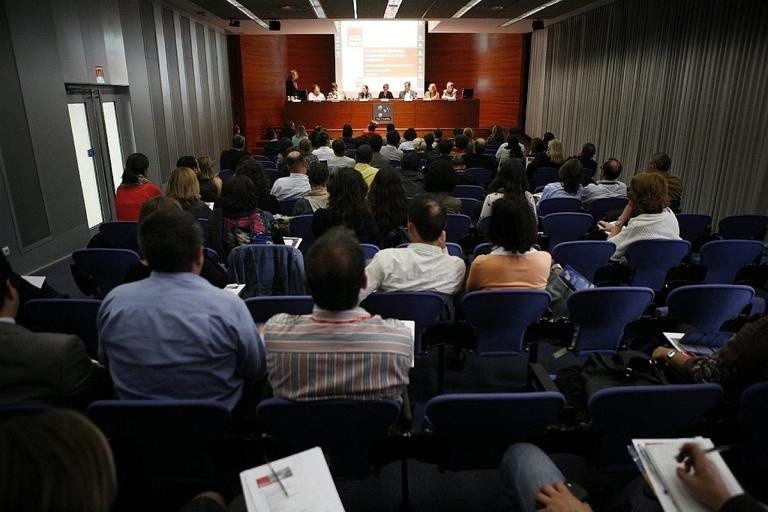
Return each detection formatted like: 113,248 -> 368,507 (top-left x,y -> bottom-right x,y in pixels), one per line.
296,89 -> 309,100
462,88 -> 473,99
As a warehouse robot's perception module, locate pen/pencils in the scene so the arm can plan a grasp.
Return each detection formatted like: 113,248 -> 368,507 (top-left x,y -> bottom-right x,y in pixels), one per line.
685,444 -> 730,465
271,465 -> 288,497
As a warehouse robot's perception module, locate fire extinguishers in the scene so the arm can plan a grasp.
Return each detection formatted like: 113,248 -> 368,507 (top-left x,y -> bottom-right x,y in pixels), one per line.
234,121 -> 241,135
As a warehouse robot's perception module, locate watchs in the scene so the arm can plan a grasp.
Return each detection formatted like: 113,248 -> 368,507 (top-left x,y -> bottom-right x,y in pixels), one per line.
666,348 -> 679,362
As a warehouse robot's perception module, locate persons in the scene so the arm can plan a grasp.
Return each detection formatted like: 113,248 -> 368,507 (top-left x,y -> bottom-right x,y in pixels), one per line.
0,247 -> 103,405
500,440 -> 768,511
326,82 -> 346,100
377,83 -> 393,100
258,224 -> 415,411
94,204 -> 268,413
306,83 -> 326,101
286,69 -> 300,101
397,81 -> 418,99
115,117 -> 686,267
652,312 -> 768,407
442,81 -> 458,103
0,400 -> 119,511
357,189 -> 466,310
463,193 -> 553,292
356,84 -> 372,99
422,83 -> 440,100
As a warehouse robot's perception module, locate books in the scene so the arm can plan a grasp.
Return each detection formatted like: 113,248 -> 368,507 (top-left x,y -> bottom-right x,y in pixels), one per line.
627,434 -> 746,511
235,444 -> 348,511
660,328 -> 736,359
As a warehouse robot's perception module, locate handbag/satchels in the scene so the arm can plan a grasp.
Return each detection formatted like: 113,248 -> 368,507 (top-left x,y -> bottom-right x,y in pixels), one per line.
553,349 -> 685,429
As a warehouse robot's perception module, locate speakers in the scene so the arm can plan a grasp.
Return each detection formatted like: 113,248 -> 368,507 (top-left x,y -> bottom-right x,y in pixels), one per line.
269,20 -> 280,30
532,20 -> 545,30
